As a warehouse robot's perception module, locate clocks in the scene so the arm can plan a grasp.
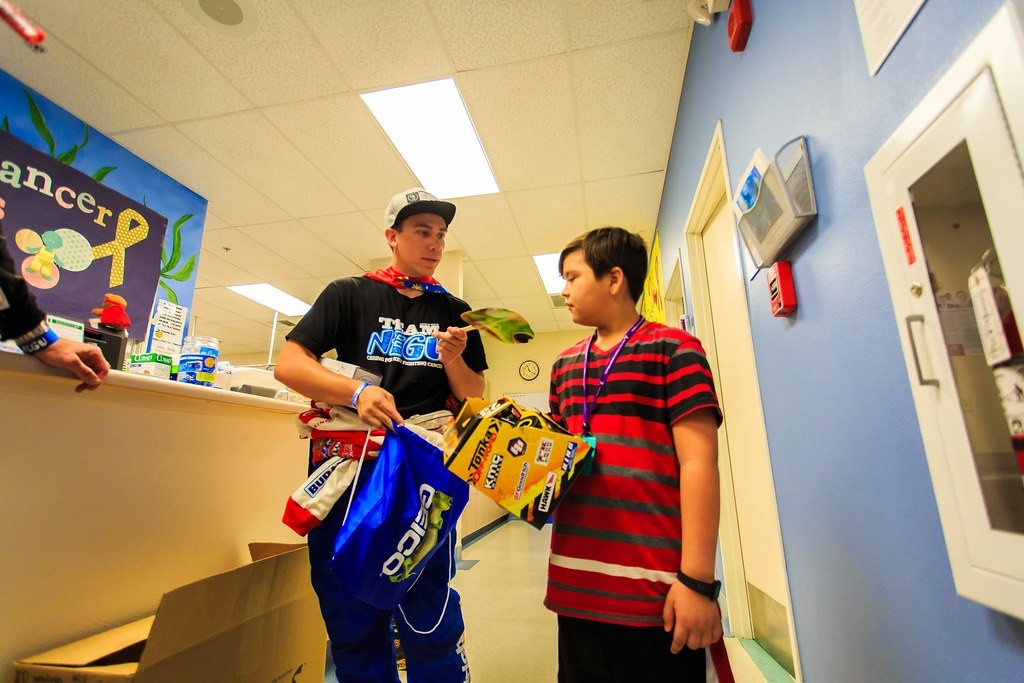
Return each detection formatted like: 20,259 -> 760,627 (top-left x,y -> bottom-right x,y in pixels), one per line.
518,360 -> 540,381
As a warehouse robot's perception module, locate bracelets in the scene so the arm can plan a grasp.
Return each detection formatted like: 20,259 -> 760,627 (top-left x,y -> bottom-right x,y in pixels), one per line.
352,382 -> 370,409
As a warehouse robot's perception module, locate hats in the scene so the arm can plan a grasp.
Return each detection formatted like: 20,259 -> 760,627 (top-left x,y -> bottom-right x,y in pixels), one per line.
384,187 -> 456,230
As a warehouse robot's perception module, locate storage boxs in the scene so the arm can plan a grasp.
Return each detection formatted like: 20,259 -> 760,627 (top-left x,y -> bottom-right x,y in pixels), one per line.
444,397 -> 594,533
11,542 -> 328,683
47,314 -> 84,342
130,353 -> 172,379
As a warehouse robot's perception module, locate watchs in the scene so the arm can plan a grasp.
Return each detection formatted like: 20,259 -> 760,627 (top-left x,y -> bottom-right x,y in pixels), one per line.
677,571 -> 721,600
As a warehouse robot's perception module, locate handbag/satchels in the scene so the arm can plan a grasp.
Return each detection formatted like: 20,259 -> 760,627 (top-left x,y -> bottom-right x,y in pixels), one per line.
318,419 -> 470,636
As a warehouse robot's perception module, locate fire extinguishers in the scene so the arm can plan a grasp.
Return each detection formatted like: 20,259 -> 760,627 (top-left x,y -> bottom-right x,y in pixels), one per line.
968,247 -> 1024,483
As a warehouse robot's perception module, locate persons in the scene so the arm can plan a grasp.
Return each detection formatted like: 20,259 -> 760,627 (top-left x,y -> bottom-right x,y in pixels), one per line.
544,227 -> 723,683
0,226 -> 112,392
275,187 -> 490,683
